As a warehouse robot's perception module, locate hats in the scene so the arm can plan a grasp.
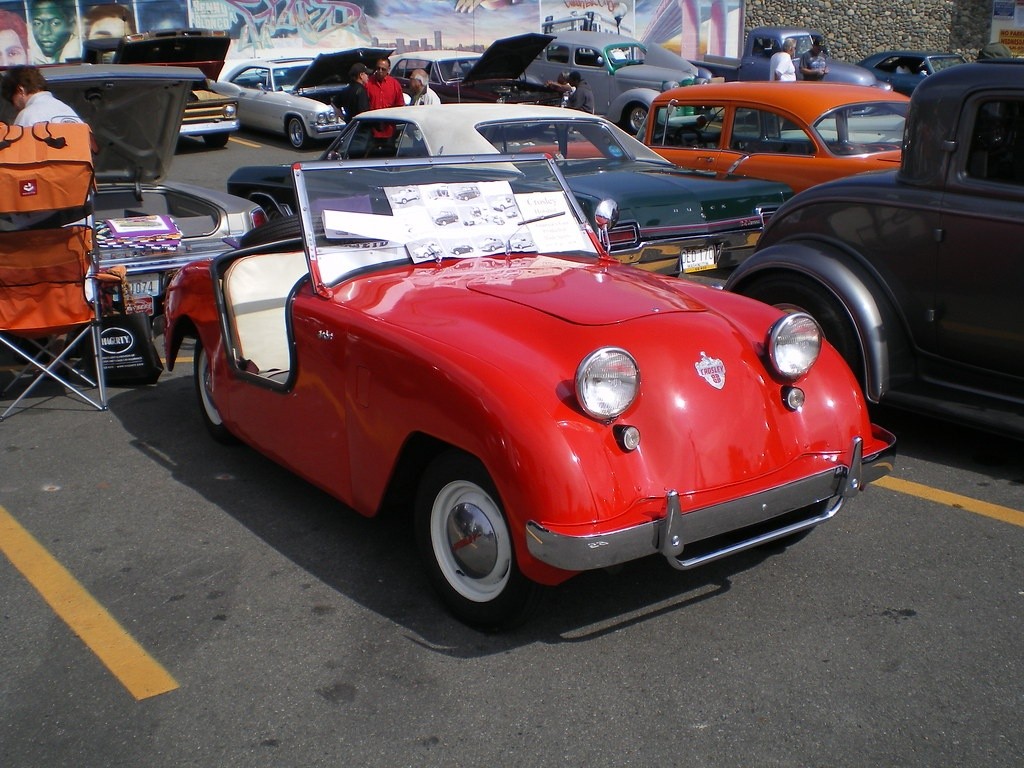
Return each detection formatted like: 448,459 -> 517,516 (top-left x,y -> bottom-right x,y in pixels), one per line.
813,38 -> 826,50
976,41 -> 1011,59
352,62 -> 374,77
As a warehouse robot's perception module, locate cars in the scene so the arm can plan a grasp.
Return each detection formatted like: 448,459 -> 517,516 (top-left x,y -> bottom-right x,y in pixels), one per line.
211,43 -> 398,151
855,49 -> 970,98
225,101 -> 795,289
384,32 -> 564,132
638,81 -> 915,197
67,27 -> 242,151
718,53 -> 1023,438
0,63 -> 272,349
519,30 -> 714,139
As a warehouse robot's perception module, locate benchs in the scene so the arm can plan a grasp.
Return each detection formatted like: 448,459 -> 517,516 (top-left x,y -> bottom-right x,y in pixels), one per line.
655,133 -> 721,146
209,236 -> 409,379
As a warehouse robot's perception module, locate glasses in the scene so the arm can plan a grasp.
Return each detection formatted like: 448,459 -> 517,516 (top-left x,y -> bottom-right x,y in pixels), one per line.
376,66 -> 388,71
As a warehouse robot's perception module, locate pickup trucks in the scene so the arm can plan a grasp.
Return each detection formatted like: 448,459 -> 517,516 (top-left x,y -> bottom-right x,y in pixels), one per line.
684,27 -> 894,93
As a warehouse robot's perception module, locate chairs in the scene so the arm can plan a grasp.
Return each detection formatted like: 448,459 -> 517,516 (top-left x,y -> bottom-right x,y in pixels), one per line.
896,65 -> 912,74
429,62 -> 448,82
752,45 -> 768,56
0,123 -> 108,418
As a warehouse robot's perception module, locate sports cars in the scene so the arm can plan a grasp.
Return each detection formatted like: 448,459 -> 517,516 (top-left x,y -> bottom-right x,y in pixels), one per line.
163,153 -> 900,638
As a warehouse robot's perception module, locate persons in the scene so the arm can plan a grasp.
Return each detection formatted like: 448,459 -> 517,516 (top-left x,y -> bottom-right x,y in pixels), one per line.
0,9 -> 29,66
85,3 -> 137,40
769,38 -> 796,139
0,64 -> 85,231
799,36 -> 830,81
364,57 -> 406,159
330,62 -> 370,125
545,71 -> 594,169
139,1 -> 186,33
31,1 -> 82,65
896,58 -> 926,74
406,69 -> 441,156
